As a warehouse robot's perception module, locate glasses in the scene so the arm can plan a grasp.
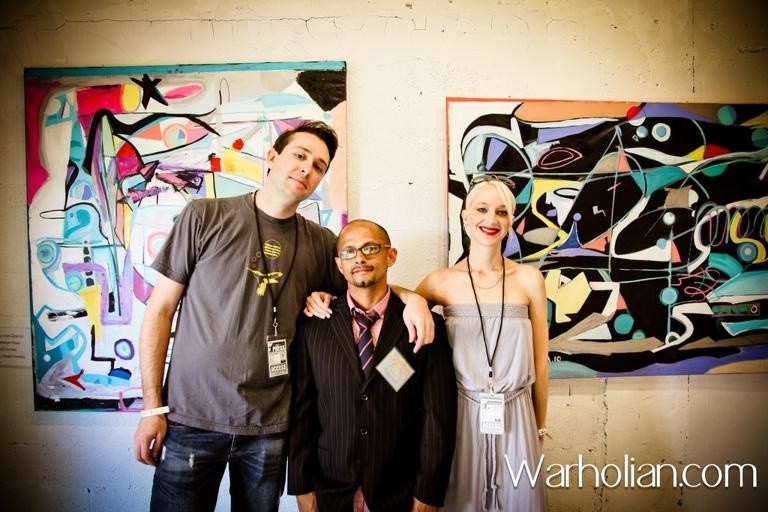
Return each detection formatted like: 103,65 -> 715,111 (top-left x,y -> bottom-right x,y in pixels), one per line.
337,244 -> 391,260
468,175 -> 517,192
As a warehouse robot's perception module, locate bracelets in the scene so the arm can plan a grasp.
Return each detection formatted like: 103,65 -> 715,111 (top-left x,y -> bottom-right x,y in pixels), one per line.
140,404 -> 172,419
536,424 -> 548,437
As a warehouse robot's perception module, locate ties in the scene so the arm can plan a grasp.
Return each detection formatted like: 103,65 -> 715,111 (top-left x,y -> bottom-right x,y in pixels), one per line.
352,308 -> 380,381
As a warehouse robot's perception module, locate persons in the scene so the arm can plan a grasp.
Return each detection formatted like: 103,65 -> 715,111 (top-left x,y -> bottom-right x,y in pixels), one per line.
132,120 -> 437,511
301,174 -> 552,511
286,219 -> 459,512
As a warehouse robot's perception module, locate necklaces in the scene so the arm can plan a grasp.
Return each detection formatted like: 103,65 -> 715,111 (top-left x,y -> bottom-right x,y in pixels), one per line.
463,256 -> 508,291
